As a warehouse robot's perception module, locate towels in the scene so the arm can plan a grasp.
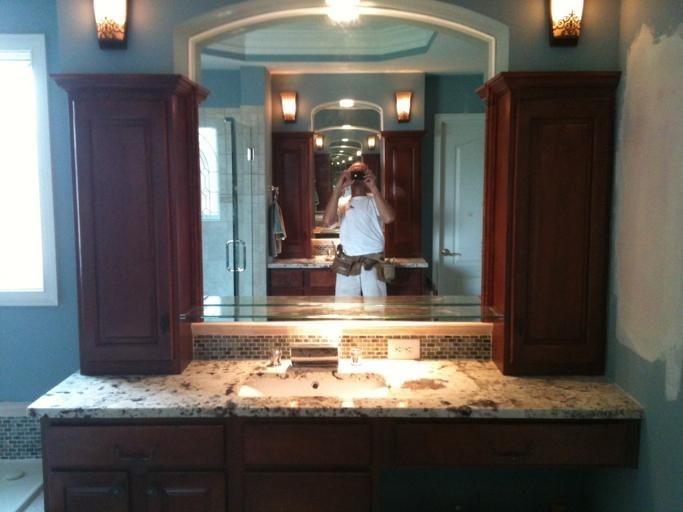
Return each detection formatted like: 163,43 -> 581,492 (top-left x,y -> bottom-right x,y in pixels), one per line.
269,201 -> 289,260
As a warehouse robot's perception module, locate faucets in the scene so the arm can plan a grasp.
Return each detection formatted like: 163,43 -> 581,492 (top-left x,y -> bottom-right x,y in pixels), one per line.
349,348 -> 361,365
269,349 -> 283,366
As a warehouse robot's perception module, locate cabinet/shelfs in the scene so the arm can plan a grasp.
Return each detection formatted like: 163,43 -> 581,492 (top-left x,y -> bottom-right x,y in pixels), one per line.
44,419 -> 228,511
395,423 -> 641,468
268,268 -> 429,297
241,418 -> 373,510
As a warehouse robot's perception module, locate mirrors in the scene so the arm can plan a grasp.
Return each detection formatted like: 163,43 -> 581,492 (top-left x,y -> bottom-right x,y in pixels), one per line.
170,0 -> 504,320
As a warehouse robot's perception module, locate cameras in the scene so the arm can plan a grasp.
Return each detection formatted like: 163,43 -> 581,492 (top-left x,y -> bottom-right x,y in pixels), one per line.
351,172 -> 365,179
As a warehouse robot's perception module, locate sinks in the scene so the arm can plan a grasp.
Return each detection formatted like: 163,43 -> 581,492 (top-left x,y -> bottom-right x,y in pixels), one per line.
237,368 -> 389,399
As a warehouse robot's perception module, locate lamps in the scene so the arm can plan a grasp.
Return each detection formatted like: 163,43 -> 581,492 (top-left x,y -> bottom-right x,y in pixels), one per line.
366,134 -> 379,150
279,86 -> 299,123
393,88 -> 414,123
314,131 -> 325,149
547,0 -> 586,47
89,0 -> 128,50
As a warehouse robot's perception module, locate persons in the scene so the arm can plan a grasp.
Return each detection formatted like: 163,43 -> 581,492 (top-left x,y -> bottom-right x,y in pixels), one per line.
322,162 -> 397,297
